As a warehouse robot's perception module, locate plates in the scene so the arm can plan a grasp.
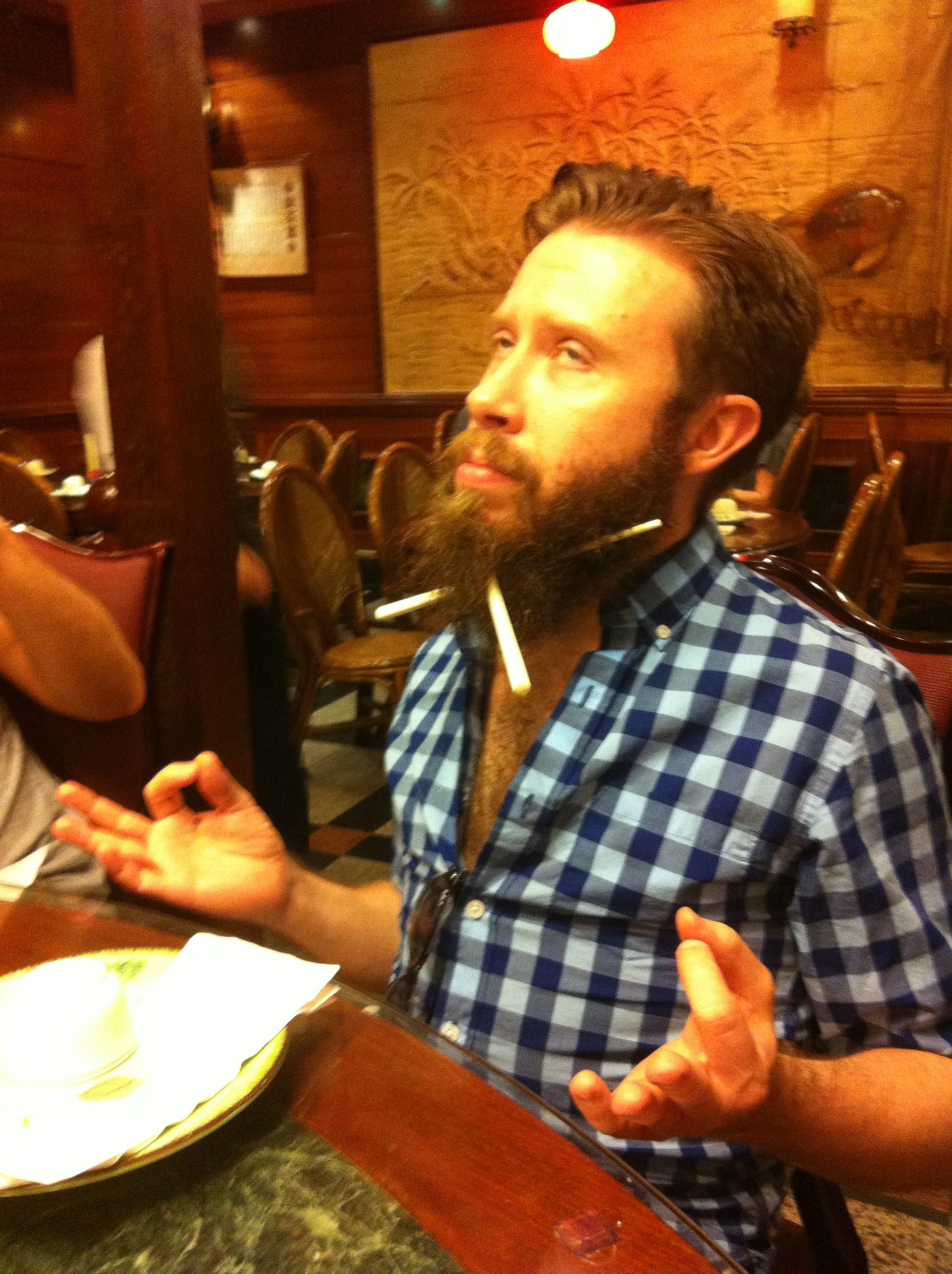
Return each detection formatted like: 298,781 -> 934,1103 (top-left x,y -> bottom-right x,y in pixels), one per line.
50,484 -> 91,496
711,511 -> 771,523
29,469 -> 58,475
1,949 -> 289,1198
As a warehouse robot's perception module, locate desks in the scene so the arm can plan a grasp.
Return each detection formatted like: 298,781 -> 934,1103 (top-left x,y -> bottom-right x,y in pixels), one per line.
0,882 -> 753,1274
36,465 -> 265,513
705,499 -> 810,557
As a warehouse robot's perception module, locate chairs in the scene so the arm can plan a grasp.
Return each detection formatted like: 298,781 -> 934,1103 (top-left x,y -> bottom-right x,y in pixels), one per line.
0,520 -> 176,813
770,413 -> 821,513
367,443 -> 445,629
882,451 -> 905,514
0,454 -> 67,542
260,462 -> 435,755
435,410 -> 461,451
866,411 -> 952,628
731,552 -> 952,766
823,474 -> 882,607
265,418 -> 335,475
319,430 -> 359,514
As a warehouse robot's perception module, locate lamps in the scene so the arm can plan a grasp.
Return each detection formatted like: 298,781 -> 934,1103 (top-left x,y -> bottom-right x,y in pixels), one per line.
770,0 -> 818,50
540,0 -> 617,60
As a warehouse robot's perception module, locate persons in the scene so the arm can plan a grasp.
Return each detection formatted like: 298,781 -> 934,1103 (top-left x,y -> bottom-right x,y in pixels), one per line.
49,161 -> 952,1274
0,519 -> 145,898
